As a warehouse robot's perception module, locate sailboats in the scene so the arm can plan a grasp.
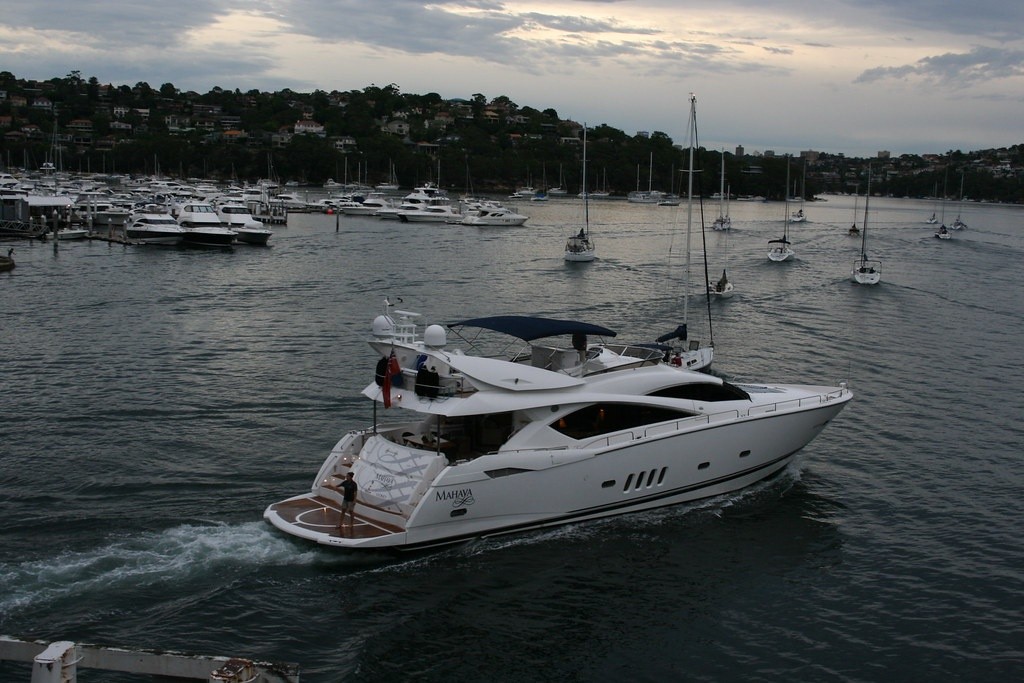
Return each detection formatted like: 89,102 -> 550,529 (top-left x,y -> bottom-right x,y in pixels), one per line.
933,168 -> 955,241
950,171 -> 971,231
577,165 -> 611,199
927,169 -> 939,224
847,182 -> 861,236
767,169 -> 798,263
709,145 -> 732,231
789,159 -> 809,222
622,91 -> 716,374
562,122 -> 598,263
782,193 -> 805,205
707,182 -> 734,299
850,161 -> 885,285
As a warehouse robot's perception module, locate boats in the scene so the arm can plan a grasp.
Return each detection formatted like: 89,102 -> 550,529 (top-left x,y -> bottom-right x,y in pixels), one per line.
263,303 -> 855,555
0,112 -> 568,249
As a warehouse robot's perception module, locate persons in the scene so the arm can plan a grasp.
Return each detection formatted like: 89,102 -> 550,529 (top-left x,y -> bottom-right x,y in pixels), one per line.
8,248 -> 14,260
709,281 -> 722,292
672,352 -> 682,365
860,265 -> 876,273
939,226 -> 948,235
332,472 -> 357,528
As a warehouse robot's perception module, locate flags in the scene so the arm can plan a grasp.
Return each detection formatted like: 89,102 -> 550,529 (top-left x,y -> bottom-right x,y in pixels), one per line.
382,345 -> 401,409
